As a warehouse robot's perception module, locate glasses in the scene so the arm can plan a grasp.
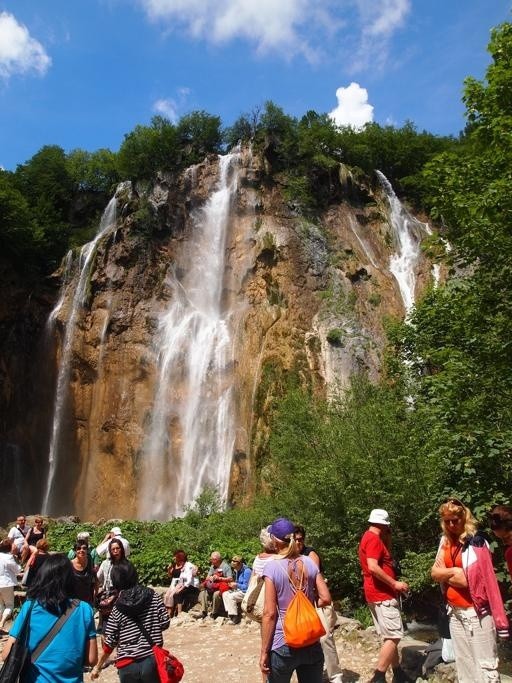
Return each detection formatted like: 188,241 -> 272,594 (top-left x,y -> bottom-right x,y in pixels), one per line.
75,546 -> 89,551
294,537 -> 303,542
443,497 -> 465,507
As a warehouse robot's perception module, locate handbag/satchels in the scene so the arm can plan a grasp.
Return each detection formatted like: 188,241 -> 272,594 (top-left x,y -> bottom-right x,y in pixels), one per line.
133,614 -> 184,683
0,599 -> 36,683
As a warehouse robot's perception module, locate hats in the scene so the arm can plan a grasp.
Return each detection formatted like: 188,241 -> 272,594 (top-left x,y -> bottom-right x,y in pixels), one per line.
110,527 -> 123,535
366,507 -> 391,526
267,517 -> 297,544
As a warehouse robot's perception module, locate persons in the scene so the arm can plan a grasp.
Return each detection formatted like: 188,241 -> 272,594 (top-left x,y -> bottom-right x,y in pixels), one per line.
24,539 -> 50,572
200,552 -> 233,620
248,525 -> 278,589
68,531 -> 101,591
94,538 -> 125,648
0,539 -> 24,633
432,497 -> 508,683
91,559 -> 184,683
222,556 -> 252,625
96,526 -> 131,558
2,553 -> 98,683
164,549 -> 200,618
359,509 -> 415,683
490,505 -> 512,583
259,518 -> 331,682
293,525 -> 344,683
8,516 -> 30,571
23,518 -> 43,572
69,539 -> 99,672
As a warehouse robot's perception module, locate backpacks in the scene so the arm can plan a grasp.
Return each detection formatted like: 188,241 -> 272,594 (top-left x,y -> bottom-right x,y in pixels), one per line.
275,554 -> 326,649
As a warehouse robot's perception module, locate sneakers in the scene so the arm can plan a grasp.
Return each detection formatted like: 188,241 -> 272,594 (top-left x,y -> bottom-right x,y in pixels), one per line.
371,675 -> 386,683
393,674 -> 415,683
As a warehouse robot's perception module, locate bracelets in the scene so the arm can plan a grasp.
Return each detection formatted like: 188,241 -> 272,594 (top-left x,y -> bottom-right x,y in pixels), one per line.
94,665 -> 102,671
